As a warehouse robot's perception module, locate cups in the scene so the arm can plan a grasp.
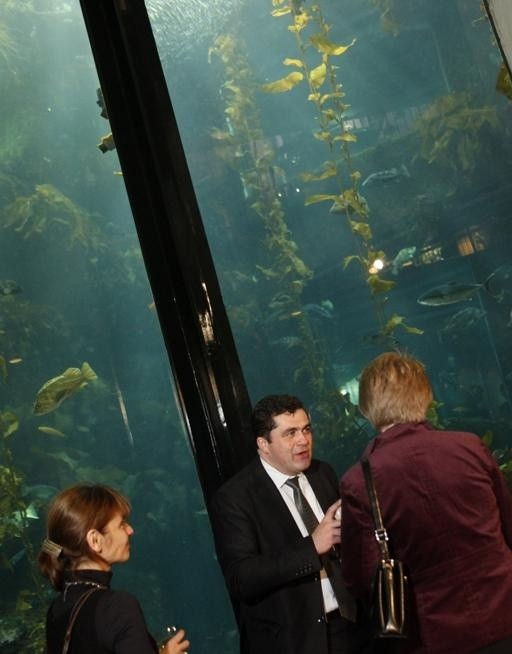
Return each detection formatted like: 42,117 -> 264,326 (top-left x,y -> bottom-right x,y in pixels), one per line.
157,624 -> 176,647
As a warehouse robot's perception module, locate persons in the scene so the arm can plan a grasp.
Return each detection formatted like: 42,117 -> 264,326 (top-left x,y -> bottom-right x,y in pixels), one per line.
338,352 -> 512,654
212,394 -> 363,654
38,485 -> 190,654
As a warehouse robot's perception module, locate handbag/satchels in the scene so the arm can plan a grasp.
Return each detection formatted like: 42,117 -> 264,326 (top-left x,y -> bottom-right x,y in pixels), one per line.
373,557 -> 413,644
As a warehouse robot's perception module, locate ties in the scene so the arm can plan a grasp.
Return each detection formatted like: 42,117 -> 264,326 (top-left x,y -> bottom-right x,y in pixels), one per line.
285,478 -> 358,623
